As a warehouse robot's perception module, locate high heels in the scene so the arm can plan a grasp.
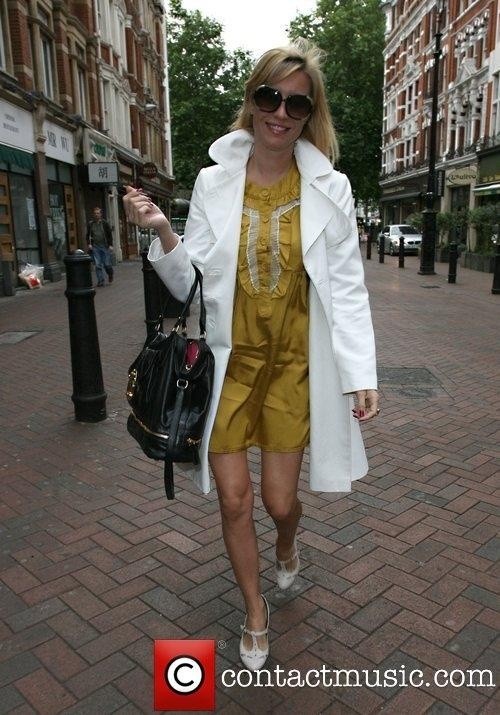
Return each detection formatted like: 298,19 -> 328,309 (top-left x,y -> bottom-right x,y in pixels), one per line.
275,532 -> 301,590
236,591 -> 272,672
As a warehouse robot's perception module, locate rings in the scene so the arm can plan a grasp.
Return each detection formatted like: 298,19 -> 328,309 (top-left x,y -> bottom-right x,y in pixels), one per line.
377,409 -> 380,412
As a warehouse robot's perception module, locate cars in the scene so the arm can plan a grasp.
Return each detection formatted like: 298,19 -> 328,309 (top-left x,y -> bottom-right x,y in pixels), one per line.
376,224 -> 423,256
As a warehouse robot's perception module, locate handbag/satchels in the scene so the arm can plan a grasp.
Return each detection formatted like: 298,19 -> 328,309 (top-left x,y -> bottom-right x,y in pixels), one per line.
127,264 -> 214,466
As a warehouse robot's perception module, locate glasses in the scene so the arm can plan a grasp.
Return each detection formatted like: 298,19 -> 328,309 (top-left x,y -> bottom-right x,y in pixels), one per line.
252,85 -> 312,119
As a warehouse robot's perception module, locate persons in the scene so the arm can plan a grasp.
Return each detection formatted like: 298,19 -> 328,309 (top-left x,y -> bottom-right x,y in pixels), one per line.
123,37 -> 383,672
87,206 -> 115,287
